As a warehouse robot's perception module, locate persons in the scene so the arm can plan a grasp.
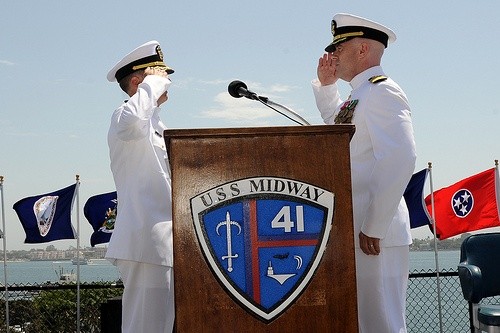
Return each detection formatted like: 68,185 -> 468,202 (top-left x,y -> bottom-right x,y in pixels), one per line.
311,13 -> 416,333
104,41 -> 174,333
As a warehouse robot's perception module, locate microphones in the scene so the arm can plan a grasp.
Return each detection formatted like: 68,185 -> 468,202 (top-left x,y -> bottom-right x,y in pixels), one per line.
227,80 -> 311,125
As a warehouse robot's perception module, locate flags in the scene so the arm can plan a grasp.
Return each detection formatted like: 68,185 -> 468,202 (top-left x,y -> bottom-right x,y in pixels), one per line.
425,166 -> 500,240
403,168 -> 432,229
13,183 -> 80,244
84,191 -> 117,247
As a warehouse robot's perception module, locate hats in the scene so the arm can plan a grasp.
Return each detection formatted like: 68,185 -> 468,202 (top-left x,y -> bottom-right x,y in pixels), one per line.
325,13 -> 397,54
106,40 -> 175,82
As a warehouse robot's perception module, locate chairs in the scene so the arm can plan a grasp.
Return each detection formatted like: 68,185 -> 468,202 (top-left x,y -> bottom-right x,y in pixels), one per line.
456,233 -> 500,333
